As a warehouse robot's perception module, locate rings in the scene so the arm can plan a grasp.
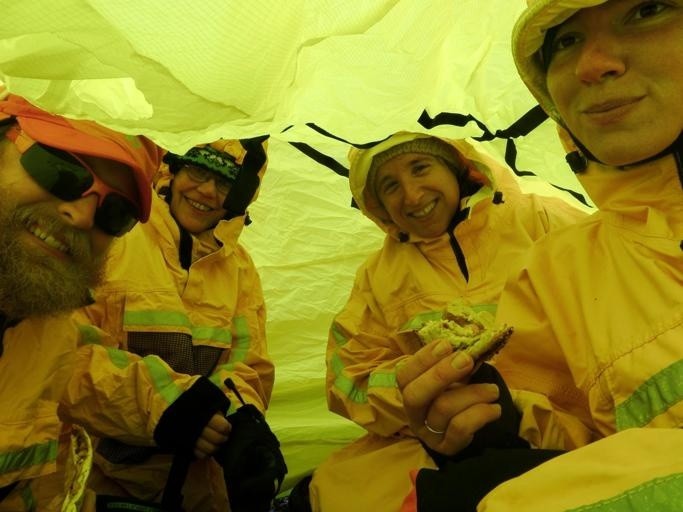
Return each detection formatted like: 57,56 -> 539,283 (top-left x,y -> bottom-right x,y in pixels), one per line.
423,420 -> 446,440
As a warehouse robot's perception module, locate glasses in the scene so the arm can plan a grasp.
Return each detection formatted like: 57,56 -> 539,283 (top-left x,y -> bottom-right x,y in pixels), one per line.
3,122 -> 145,238
180,160 -> 234,196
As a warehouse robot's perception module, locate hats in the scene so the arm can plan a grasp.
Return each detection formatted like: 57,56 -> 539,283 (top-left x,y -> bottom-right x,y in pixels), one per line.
174,145 -> 242,179
2,94 -> 164,223
366,137 -> 461,201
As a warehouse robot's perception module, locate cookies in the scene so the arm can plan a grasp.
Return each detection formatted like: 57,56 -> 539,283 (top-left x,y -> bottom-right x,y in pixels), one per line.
56,421 -> 93,511
411,304 -> 514,382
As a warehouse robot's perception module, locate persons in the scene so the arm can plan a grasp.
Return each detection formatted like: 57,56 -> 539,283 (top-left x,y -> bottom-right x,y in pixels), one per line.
393,0 -> 683,510
80,134 -> 288,511
284,132 -> 596,511
0,78 -> 167,512
